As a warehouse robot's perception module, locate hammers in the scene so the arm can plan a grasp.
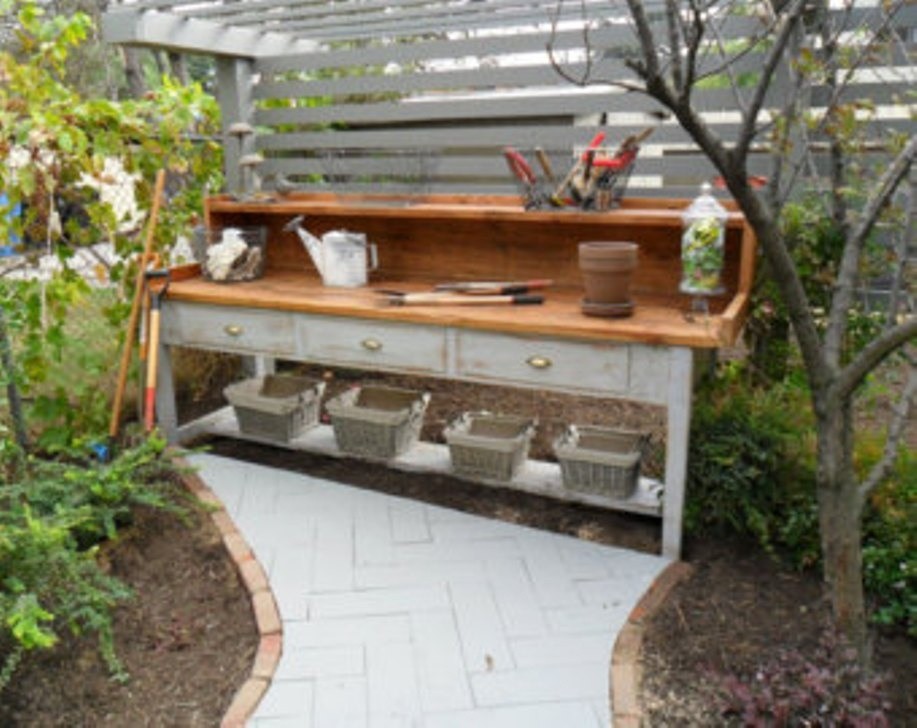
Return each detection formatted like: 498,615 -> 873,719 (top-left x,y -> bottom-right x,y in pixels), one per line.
539,131 -> 605,210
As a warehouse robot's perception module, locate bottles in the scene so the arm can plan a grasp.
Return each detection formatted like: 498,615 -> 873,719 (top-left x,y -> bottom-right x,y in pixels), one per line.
678,182 -> 727,296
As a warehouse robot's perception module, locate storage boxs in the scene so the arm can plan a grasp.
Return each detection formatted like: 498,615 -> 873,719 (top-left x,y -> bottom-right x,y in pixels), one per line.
223,376 -> 326,439
326,383 -> 432,456
555,425 -> 654,496
442,411 -> 538,481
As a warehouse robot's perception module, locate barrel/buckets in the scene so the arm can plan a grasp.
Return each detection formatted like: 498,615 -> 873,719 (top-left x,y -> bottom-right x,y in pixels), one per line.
579,241 -> 638,303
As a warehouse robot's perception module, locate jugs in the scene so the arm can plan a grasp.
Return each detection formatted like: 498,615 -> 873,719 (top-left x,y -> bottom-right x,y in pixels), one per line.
281,215 -> 378,289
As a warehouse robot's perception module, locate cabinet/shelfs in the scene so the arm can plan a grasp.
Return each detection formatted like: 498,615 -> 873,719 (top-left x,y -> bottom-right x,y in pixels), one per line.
146,195 -> 763,560
